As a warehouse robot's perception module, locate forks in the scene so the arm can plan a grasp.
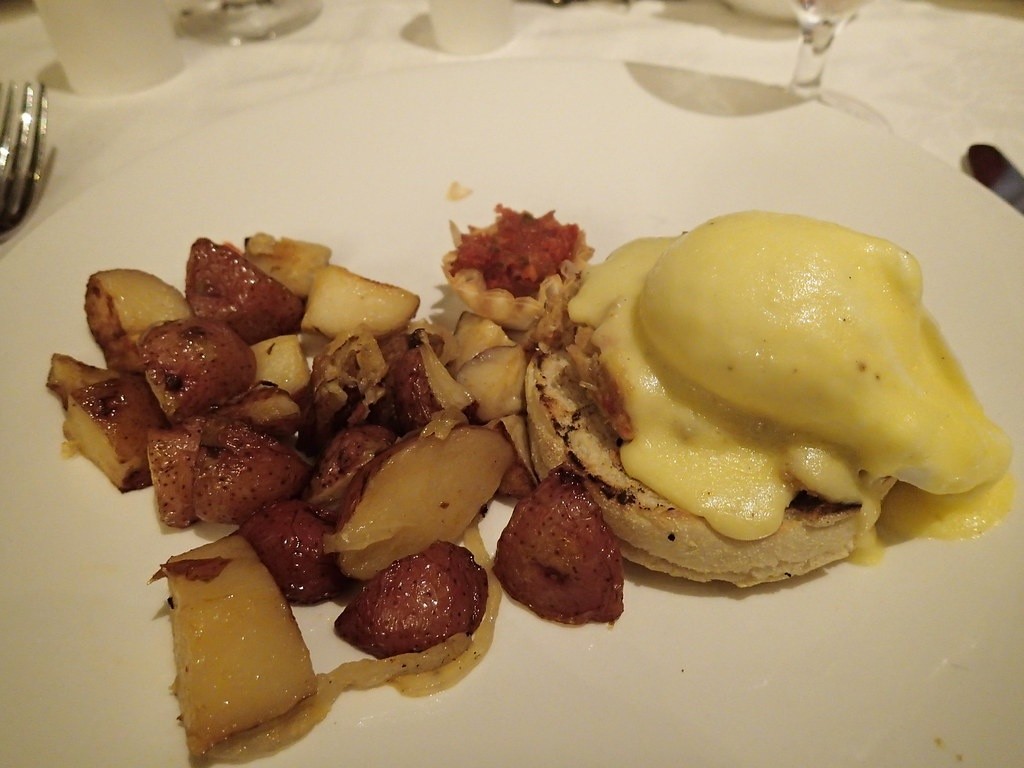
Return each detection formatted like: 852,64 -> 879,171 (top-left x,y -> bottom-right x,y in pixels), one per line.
0,80 -> 48,236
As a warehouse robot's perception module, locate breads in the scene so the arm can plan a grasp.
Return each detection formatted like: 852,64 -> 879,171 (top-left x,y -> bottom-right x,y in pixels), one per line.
522,331 -> 900,588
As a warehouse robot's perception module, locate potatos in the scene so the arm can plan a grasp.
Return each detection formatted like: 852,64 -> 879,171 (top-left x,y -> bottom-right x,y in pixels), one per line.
50,232 -> 623,765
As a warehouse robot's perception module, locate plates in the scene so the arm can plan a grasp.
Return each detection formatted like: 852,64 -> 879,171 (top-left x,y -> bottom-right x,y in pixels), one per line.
0,59 -> 1024,768
